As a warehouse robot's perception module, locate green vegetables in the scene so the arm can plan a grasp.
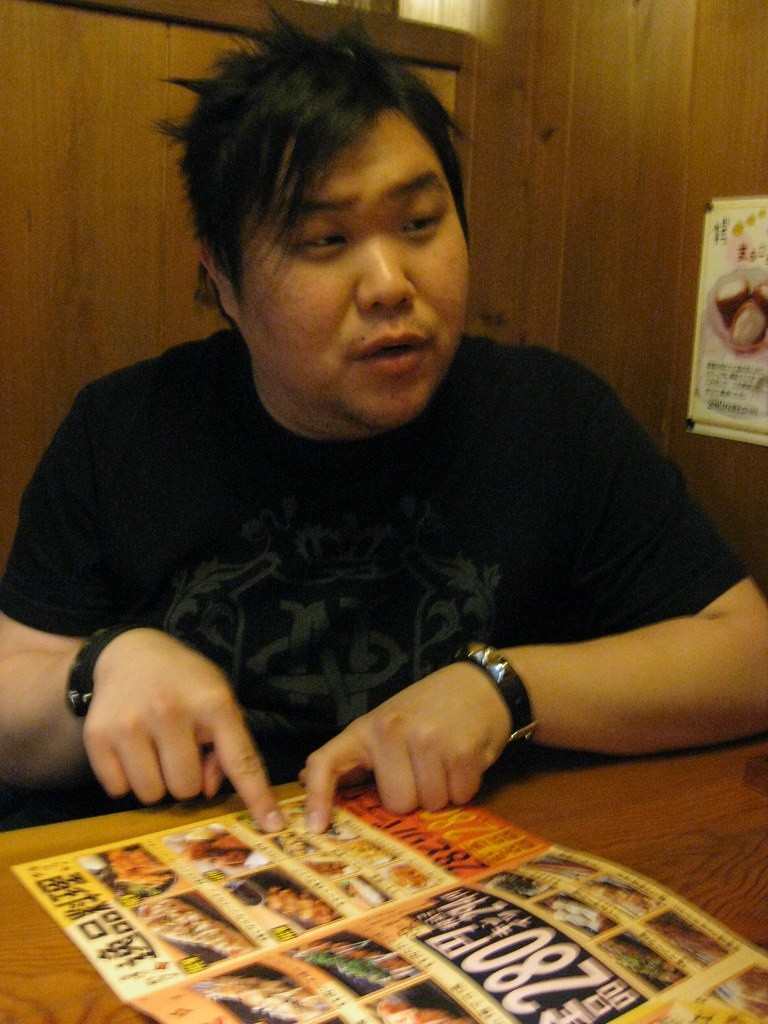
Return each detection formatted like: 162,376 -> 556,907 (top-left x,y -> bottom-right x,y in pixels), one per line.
304,952 -> 390,981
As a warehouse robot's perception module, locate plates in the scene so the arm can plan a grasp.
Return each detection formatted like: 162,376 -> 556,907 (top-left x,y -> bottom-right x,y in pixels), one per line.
88,824 -> 767,1024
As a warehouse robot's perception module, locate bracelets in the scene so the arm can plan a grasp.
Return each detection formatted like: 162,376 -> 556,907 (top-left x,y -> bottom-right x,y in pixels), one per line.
64,619 -> 161,719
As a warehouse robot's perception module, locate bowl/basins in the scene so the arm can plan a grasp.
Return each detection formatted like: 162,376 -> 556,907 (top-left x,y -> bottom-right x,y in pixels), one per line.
704,266 -> 768,357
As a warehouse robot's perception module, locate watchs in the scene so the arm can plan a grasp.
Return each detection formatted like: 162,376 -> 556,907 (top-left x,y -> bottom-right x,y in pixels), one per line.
452,642 -> 539,746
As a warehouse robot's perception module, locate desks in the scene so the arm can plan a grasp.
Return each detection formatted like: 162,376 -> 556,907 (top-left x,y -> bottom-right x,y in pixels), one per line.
0,737 -> 768,1024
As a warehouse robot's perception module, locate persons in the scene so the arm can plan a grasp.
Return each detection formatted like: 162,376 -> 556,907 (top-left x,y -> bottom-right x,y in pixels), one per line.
2,16 -> 768,830
